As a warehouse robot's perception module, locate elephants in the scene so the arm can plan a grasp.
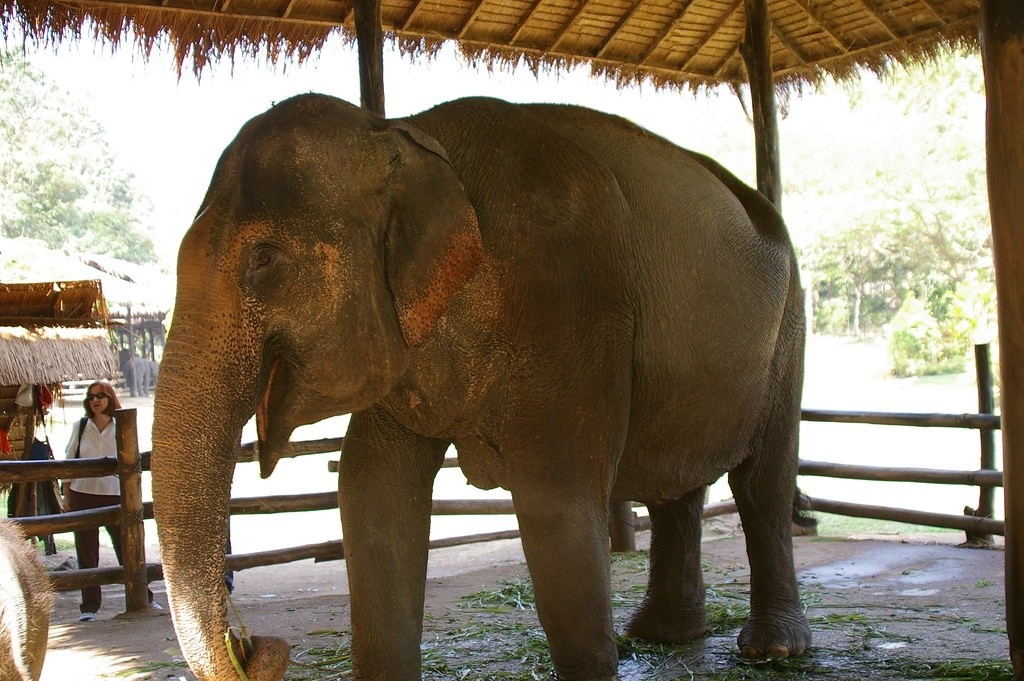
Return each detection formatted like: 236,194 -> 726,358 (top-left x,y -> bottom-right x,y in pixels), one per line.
150,92 -> 813,681
0,521 -> 52,681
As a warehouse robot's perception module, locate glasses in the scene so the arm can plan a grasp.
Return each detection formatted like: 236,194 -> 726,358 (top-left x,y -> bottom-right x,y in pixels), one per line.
87,392 -> 108,400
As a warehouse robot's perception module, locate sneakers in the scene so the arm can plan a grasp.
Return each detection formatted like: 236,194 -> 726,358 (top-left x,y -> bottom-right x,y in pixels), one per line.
79,611 -> 96,622
149,601 -> 162,610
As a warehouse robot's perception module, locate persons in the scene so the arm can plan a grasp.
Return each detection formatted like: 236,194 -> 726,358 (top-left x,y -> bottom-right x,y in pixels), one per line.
61,380 -> 163,622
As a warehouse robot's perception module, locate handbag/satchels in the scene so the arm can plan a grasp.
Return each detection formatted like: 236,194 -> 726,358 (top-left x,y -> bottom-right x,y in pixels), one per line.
31,439 -> 52,461
